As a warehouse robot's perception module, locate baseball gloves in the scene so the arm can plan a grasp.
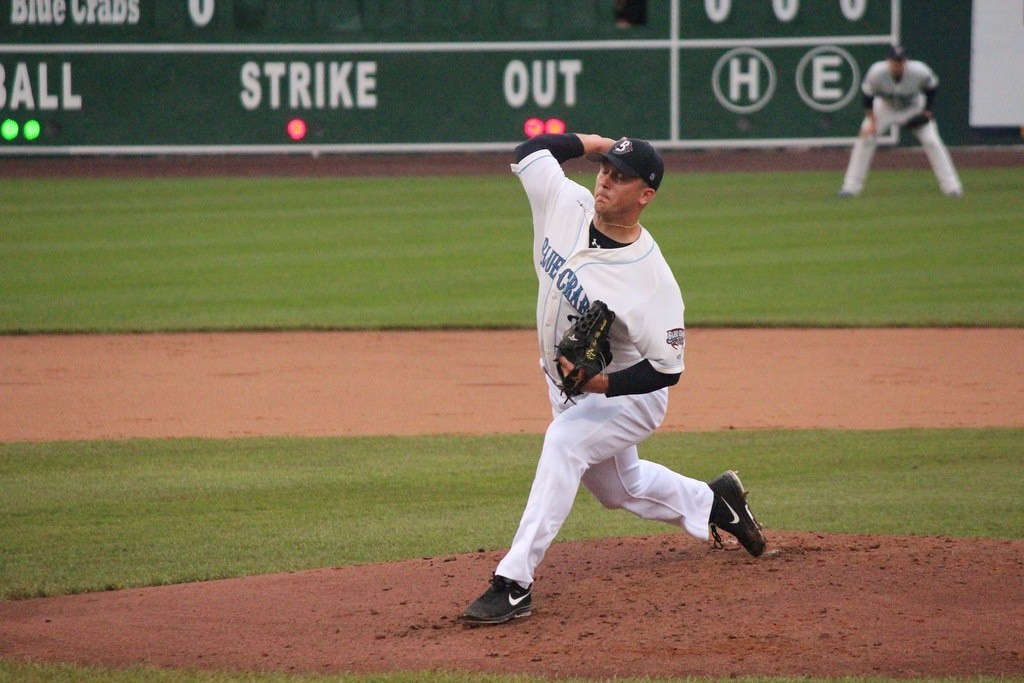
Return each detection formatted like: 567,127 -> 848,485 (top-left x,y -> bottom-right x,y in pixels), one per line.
554,299 -> 615,396
901,114 -> 929,130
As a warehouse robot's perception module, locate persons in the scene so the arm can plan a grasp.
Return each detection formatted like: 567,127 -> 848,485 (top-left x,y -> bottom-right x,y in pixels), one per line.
835,47 -> 963,195
462,133 -> 766,623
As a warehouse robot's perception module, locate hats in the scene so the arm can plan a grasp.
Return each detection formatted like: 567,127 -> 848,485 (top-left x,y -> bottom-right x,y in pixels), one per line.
586,139 -> 664,193
888,44 -> 905,60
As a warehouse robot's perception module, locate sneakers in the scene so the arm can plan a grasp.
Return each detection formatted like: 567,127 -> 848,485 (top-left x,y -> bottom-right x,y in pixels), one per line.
462,571 -> 532,624
708,469 -> 765,557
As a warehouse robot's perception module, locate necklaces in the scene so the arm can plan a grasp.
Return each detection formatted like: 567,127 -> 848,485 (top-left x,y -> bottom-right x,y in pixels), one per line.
578,200 -> 639,228
